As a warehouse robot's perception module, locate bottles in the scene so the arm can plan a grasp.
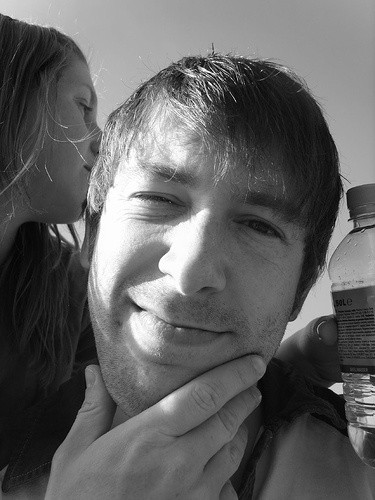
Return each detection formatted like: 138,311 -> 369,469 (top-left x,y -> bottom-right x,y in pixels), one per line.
327,183 -> 375,469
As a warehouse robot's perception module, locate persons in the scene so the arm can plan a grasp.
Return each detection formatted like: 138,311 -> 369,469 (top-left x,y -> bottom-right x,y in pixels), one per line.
0,14 -> 345,500
0,50 -> 375,500
